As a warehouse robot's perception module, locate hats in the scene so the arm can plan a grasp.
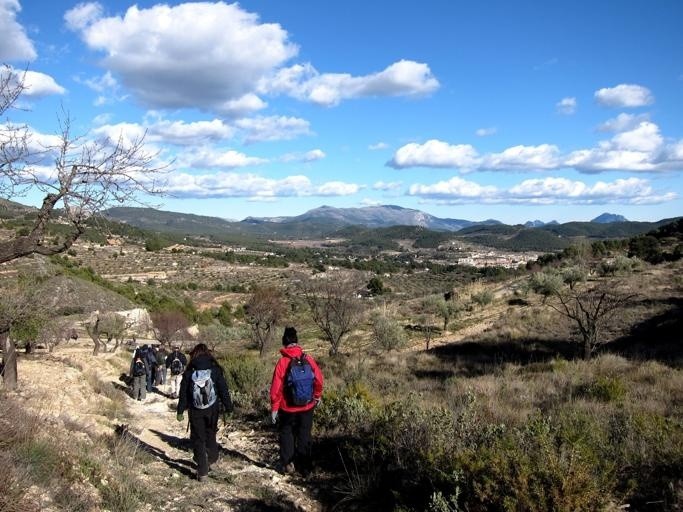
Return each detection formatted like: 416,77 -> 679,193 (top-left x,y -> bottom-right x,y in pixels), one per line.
283,326 -> 297,345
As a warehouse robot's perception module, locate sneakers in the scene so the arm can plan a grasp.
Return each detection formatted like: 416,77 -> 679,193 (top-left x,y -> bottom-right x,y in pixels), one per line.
282,461 -> 315,480
196,468 -> 208,482
169,392 -> 180,398
133,382 -> 164,402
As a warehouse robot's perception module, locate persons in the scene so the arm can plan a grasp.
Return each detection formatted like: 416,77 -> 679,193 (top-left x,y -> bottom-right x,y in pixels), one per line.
269,324 -> 324,477
128,344 -> 188,400
175,342 -> 234,482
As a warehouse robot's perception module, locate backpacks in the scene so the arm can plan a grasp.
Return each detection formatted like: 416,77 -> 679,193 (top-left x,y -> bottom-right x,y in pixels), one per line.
281,358 -> 314,406
191,369 -> 218,409
132,359 -> 144,376
171,359 -> 181,375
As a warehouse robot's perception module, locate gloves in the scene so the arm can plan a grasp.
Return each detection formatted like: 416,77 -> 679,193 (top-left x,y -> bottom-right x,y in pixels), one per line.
175,414 -> 185,421
315,398 -> 320,409
271,411 -> 279,424
223,412 -> 233,420
